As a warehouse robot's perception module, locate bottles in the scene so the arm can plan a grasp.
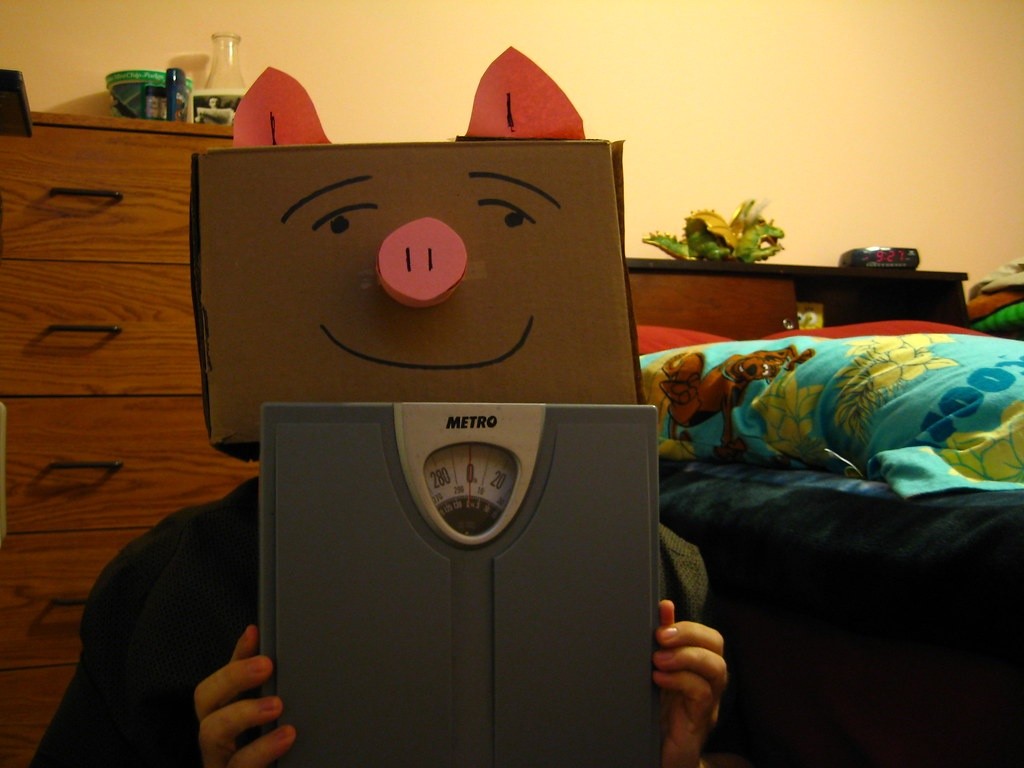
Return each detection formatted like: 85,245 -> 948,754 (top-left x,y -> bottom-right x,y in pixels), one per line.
205,31 -> 247,91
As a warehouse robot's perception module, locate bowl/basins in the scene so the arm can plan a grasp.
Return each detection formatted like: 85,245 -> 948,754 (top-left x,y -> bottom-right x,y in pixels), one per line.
105,70 -> 193,122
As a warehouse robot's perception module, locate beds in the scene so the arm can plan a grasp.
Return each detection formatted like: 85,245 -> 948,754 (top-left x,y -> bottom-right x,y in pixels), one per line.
629,246 -> 1024,768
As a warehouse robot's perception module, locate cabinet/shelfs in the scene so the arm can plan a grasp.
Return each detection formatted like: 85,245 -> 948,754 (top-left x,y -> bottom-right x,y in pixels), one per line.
0,109 -> 257,768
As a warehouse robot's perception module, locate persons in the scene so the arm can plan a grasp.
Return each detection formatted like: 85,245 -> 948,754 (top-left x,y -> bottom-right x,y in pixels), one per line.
195,97 -> 235,125
30,478 -> 726,768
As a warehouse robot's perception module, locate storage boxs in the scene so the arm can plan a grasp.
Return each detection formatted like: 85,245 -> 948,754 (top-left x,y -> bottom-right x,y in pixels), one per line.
190,137 -> 648,463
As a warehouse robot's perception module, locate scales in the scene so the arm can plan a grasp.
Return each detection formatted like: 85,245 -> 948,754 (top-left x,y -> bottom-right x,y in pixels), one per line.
254,402 -> 664,768
